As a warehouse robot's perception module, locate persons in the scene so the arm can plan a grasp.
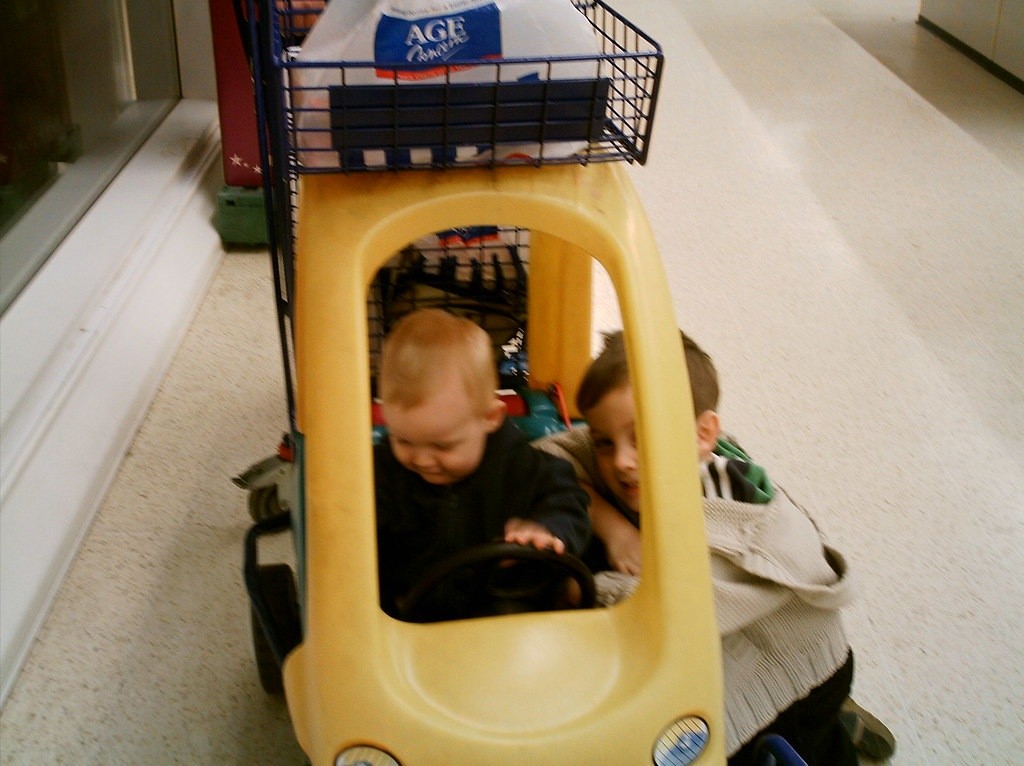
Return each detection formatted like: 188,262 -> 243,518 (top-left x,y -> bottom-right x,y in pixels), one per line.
528,330 -> 896,766
373,310 -> 590,624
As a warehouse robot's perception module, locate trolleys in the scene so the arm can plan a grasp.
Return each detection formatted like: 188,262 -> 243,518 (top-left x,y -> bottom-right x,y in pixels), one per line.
233,0 -> 806,763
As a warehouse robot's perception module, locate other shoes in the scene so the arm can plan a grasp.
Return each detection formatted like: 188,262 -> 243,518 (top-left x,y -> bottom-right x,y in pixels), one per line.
839,696 -> 895,761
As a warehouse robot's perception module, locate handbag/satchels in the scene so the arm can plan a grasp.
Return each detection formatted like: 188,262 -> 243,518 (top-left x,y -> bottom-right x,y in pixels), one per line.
290,1 -> 603,167
